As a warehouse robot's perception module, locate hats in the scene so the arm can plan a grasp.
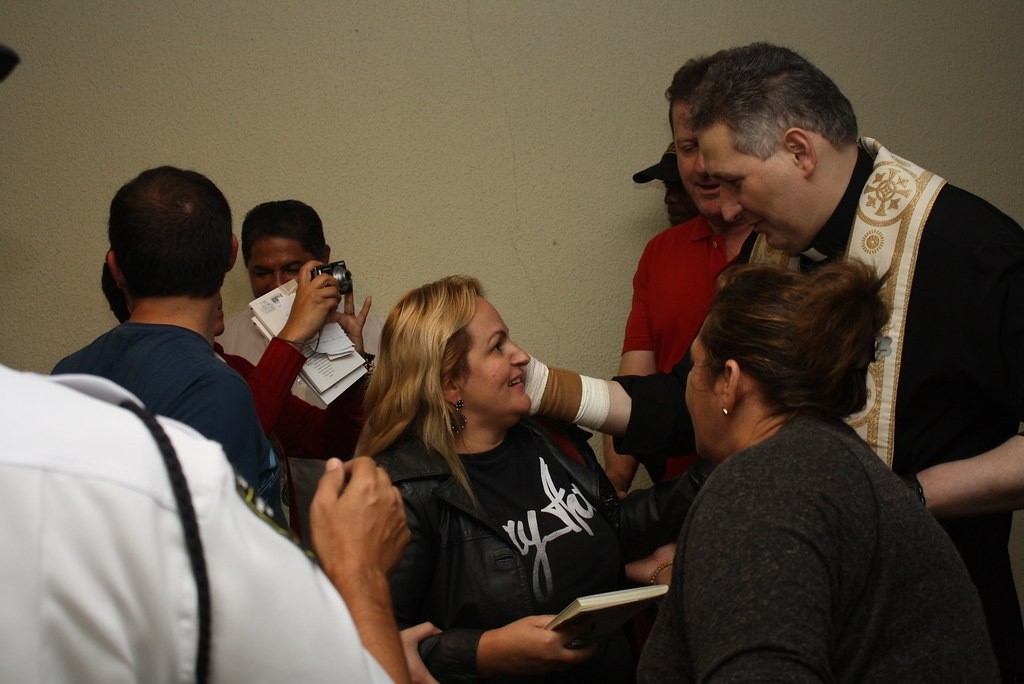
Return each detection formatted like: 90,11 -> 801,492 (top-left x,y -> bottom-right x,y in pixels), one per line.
633,142 -> 682,188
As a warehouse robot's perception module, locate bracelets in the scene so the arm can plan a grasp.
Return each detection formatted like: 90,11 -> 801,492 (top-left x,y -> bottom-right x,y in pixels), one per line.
650,561 -> 673,585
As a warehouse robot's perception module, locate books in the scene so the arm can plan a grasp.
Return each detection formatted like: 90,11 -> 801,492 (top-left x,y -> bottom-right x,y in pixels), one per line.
544,585 -> 669,648
249,279 -> 368,405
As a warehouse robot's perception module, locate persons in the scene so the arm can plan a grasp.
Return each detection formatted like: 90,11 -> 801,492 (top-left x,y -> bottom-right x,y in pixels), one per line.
357,275 -> 658,684
633,142 -> 699,226
51,166 -> 440,684
626,262 -> 1001,684
522,43 -> 1024,684
101,260 -> 371,541
217,199 -> 386,544
0,364 -> 412,684
602,55 -> 754,499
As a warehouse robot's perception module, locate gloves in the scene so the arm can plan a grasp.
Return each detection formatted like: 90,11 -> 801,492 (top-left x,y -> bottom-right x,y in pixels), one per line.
520,354 -> 609,431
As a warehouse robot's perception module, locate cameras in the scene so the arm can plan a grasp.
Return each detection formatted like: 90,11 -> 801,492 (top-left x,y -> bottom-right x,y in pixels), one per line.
311,260 -> 353,295
